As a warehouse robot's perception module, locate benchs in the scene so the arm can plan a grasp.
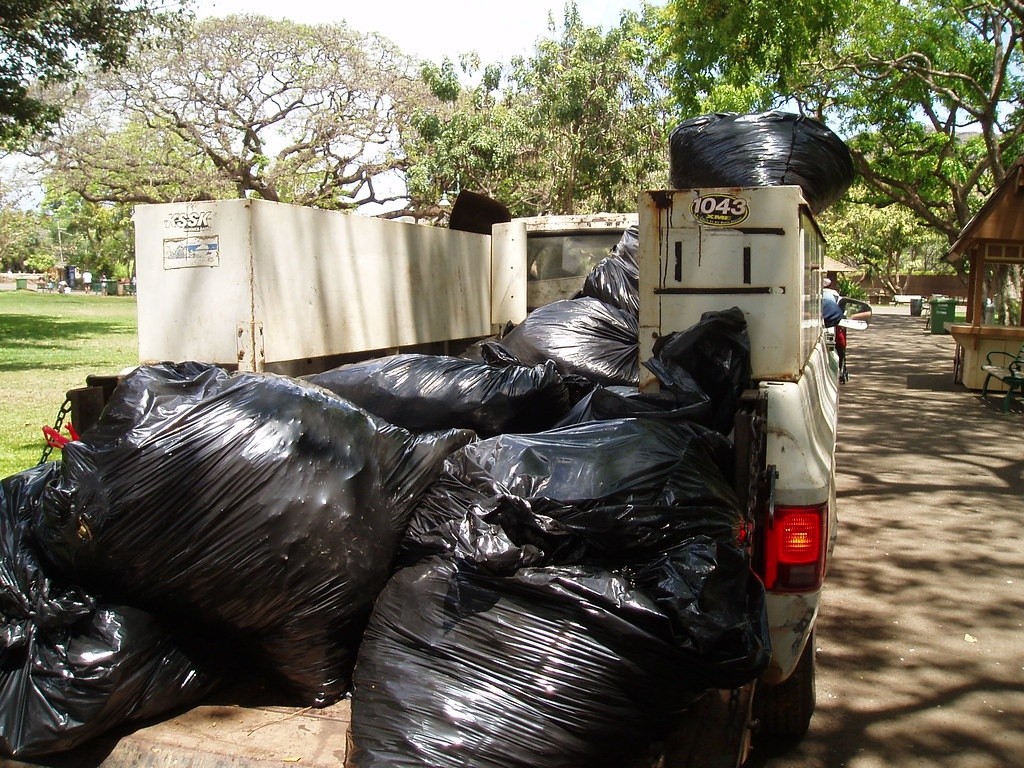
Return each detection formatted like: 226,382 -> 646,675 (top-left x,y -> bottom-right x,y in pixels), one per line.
980,342 -> 1024,413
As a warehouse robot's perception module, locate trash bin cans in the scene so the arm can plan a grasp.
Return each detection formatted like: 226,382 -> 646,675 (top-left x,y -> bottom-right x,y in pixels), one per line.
911,299 -> 922,317
929,298 -> 957,335
106,280 -> 117,296
16,279 -> 27,290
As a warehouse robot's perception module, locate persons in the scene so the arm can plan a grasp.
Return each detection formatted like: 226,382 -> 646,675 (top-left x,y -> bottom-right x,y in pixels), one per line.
822,279 -> 844,328
49,275 -> 56,292
83,269 -> 92,293
100,272 -> 108,295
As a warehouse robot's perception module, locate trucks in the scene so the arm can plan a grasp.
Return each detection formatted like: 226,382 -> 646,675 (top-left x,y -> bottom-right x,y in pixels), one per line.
132,182 -> 871,768
